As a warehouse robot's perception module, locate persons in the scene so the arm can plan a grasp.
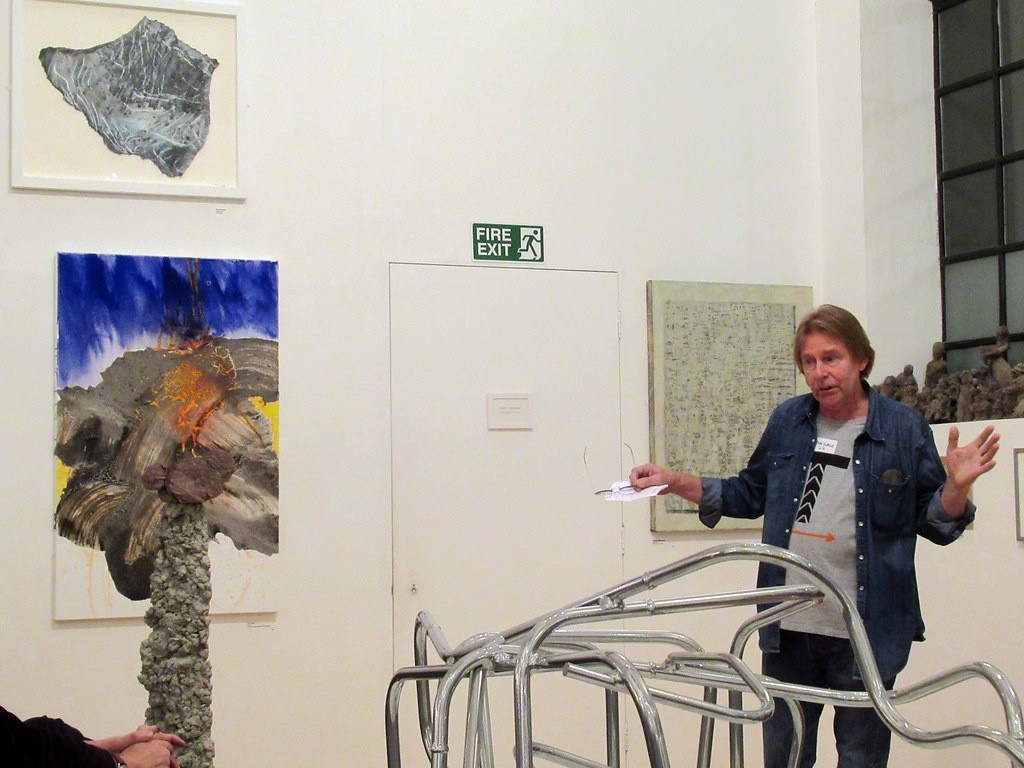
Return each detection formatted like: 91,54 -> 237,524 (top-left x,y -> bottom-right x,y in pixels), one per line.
1,706 -> 186,768
630,303 -> 1001,768
